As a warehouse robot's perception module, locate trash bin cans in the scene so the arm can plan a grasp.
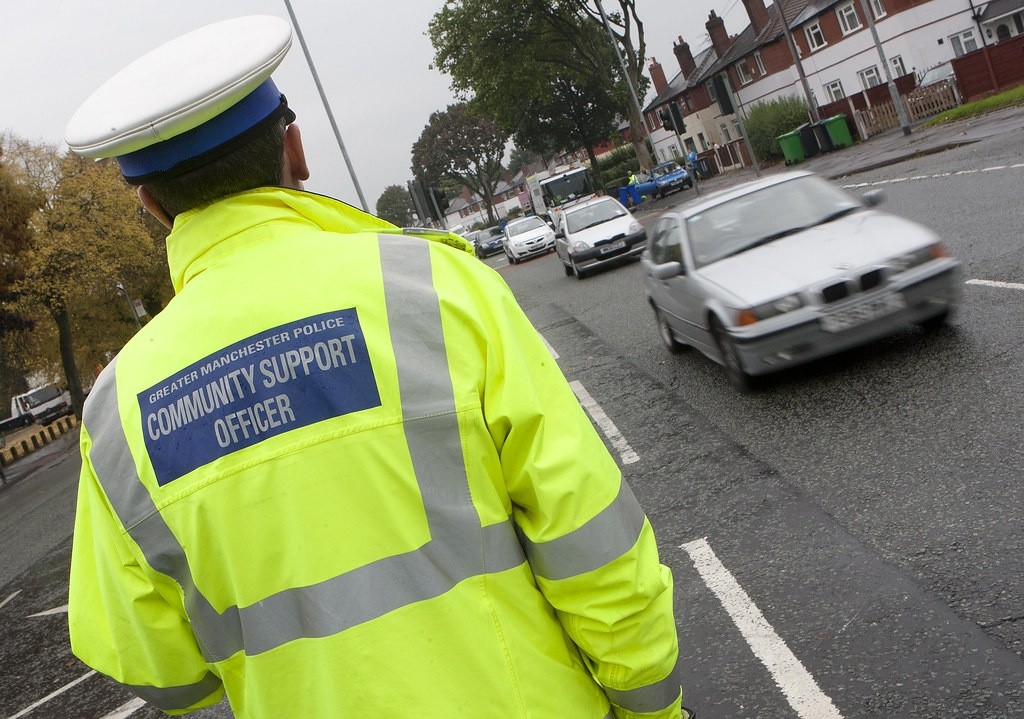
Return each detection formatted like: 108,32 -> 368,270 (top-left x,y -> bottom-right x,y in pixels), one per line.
619,187 -> 628,204
692,156 -> 715,180
608,185 -> 618,200
628,184 -> 643,205
776,113 -> 854,166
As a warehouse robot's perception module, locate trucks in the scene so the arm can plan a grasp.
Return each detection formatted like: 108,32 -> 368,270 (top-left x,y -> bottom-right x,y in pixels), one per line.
22,361 -> 93,427
0,393 -> 33,431
517,158 -> 599,233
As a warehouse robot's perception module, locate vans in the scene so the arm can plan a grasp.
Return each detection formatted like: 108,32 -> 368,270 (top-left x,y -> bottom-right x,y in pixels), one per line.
449,224 -> 470,237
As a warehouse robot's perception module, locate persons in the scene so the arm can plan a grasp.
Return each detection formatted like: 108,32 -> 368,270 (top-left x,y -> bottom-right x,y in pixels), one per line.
711,142 -> 720,150
628,171 -> 646,200
63,13 -> 694,719
640,165 -> 657,201
687,147 -> 704,181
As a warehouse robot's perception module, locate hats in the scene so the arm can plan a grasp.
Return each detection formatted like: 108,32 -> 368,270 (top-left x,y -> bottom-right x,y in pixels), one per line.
63,16 -> 296,185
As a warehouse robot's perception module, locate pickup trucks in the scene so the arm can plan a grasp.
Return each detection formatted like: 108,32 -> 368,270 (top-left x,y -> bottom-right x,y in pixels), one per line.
635,159 -> 693,200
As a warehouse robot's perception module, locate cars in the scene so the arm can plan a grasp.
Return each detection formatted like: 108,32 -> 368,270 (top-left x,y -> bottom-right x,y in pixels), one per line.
641,170 -> 962,392
463,230 -> 481,246
554,196 -> 648,280
501,215 -> 558,265
474,226 -> 506,259
